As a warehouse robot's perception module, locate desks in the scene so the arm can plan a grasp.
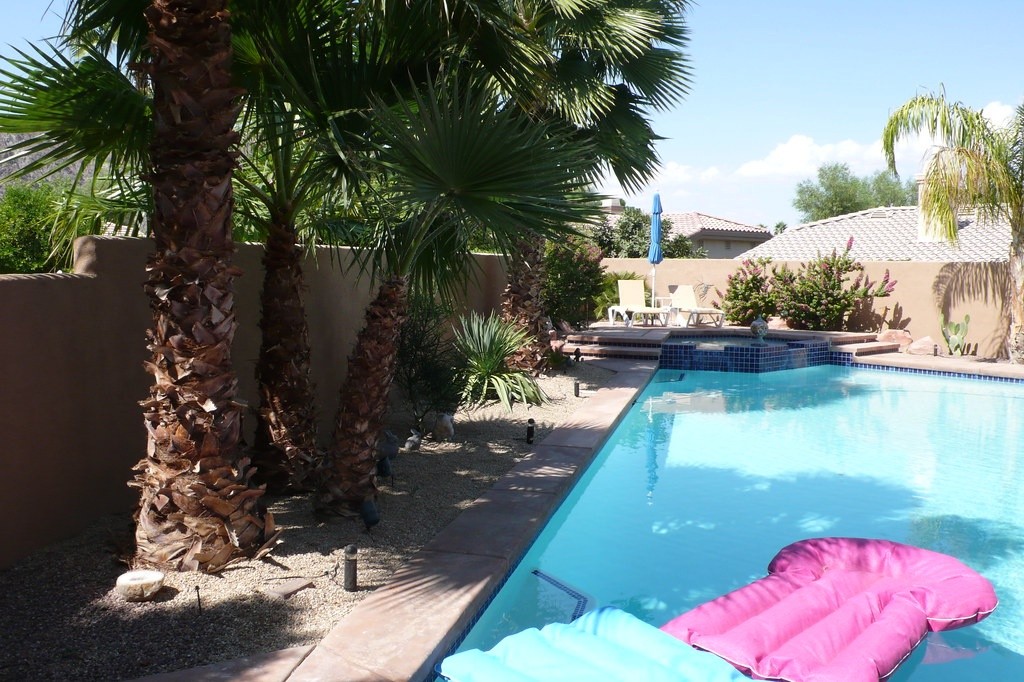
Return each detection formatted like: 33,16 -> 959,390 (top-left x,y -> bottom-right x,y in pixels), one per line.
665,306 -> 682,326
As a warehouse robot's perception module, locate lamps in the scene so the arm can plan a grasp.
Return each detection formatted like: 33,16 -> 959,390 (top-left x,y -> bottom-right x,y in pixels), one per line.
525,418 -> 535,445
573,376 -> 580,397
933,344 -> 938,358
341,543 -> 358,592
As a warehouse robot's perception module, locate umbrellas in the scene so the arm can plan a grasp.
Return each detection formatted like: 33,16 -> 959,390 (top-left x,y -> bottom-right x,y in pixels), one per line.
648,192 -> 663,327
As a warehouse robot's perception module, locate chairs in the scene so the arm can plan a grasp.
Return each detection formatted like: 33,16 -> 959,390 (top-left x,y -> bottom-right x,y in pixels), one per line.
667,284 -> 725,328
608,280 -> 670,328
